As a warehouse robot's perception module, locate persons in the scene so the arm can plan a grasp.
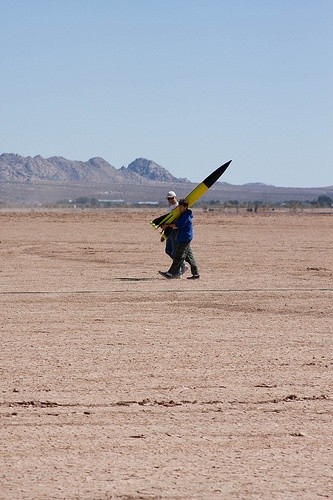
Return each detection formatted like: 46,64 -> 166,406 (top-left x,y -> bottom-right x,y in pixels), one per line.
165,190 -> 189,279
158,199 -> 200,279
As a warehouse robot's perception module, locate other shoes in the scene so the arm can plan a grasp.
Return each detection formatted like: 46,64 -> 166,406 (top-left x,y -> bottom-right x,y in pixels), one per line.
187,275 -> 199,279
174,273 -> 182,279
158,271 -> 172,278
181,265 -> 189,273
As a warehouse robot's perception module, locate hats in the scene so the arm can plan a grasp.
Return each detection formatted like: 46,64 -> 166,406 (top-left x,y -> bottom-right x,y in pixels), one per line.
166,191 -> 176,198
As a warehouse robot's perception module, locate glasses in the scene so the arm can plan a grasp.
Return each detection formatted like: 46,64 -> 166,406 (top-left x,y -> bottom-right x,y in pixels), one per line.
167,197 -> 175,200
